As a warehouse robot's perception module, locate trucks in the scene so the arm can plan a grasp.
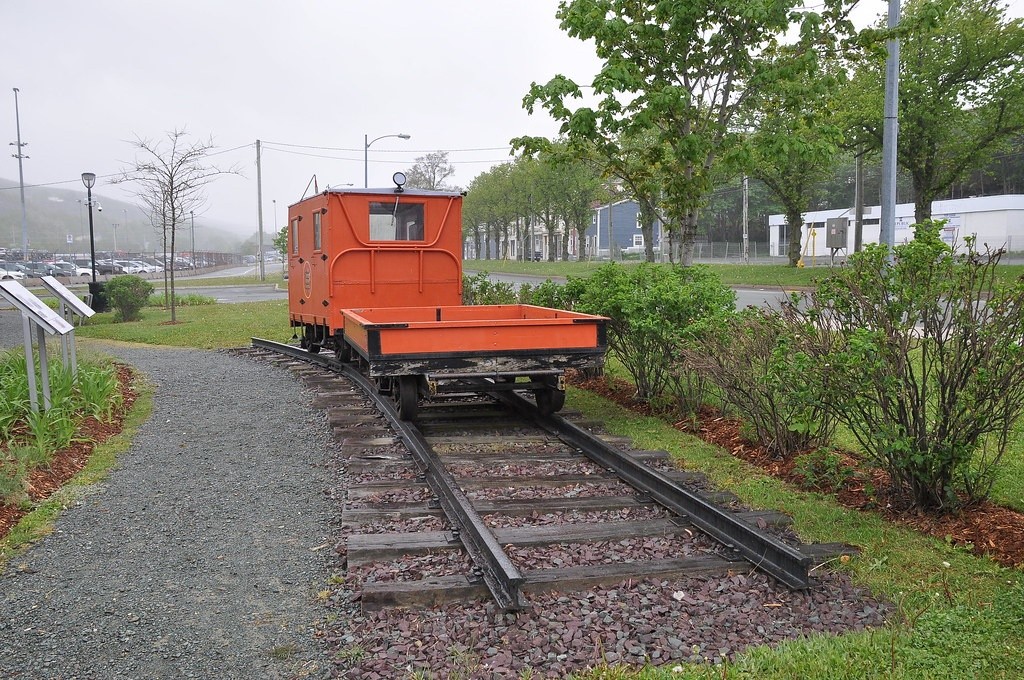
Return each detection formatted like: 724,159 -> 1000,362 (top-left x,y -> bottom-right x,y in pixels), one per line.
287,173 -> 611,421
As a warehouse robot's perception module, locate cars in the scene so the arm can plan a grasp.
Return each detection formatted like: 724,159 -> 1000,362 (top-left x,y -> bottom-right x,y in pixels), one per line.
0,248 -> 215,280
243,249 -> 288,262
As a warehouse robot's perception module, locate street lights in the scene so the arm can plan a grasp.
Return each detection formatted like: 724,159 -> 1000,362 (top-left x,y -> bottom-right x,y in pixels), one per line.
82,172 -> 96,282
365,133 -> 411,189
